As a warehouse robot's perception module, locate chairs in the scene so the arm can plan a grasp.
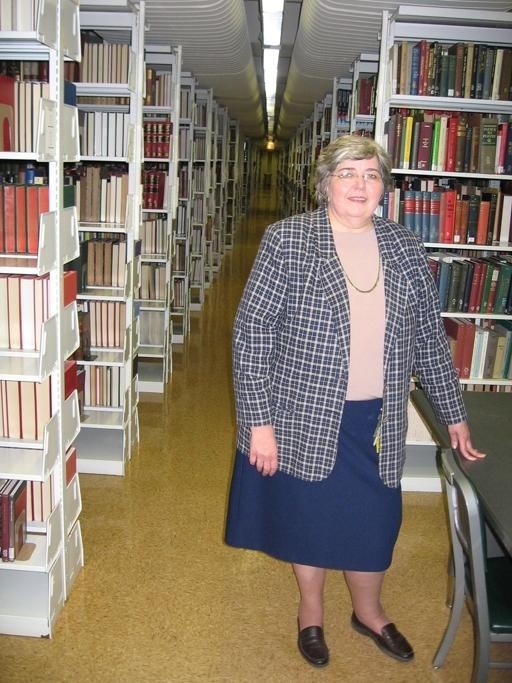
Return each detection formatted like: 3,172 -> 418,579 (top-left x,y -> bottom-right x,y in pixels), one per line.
432,446 -> 510,683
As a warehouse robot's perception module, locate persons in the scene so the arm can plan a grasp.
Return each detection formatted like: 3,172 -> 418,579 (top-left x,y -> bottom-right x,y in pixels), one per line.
223,135 -> 486,665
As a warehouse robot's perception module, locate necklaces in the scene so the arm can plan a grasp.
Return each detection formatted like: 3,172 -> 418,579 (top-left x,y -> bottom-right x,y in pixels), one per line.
337,253 -> 381,293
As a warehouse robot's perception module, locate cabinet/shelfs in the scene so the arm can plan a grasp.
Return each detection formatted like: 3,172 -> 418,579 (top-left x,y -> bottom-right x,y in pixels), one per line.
0,2 -> 86,641
272,2 -> 512,500
71,0 -> 146,475
136,43 -> 263,394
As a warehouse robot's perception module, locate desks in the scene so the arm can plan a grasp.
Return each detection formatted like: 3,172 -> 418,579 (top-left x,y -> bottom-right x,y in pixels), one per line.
403,383 -> 512,565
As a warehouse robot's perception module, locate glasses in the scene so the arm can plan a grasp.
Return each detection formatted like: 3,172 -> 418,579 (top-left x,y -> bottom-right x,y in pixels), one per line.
331,171 -> 381,184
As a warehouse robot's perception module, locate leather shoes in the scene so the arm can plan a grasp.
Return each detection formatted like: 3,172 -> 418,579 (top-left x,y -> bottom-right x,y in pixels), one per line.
351,610 -> 414,662
297,616 -> 329,667
